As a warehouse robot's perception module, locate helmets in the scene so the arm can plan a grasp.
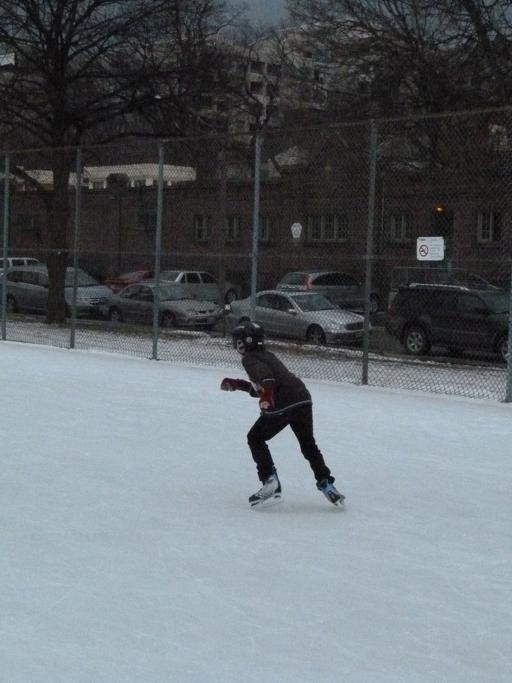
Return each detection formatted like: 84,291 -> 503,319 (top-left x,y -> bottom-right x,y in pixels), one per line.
231,321 -> 265,355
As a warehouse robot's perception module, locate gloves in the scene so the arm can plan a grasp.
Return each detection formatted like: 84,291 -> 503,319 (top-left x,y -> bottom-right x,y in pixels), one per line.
220,377 -> 251,392
259,384 -> 275,409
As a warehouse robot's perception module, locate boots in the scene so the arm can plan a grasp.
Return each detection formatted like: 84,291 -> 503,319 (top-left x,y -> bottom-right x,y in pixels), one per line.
249,472 -> 282,505
317,479 -> 344,503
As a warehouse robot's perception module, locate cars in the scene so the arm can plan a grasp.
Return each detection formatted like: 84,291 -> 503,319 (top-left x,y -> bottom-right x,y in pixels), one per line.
224,288 -> 374,346
2,263 -> 114,318
97,281 -> 224,332
155,270 -> 242,305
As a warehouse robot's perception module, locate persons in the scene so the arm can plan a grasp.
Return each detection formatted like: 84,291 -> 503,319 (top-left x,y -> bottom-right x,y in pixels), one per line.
221,321 -> 345,506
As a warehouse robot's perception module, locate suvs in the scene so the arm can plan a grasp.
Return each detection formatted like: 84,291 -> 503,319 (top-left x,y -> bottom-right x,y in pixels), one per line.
382,265 -> 505,308
382,281 -> 512,365
276,269 -> 384,317
0,256 -> 42,275
